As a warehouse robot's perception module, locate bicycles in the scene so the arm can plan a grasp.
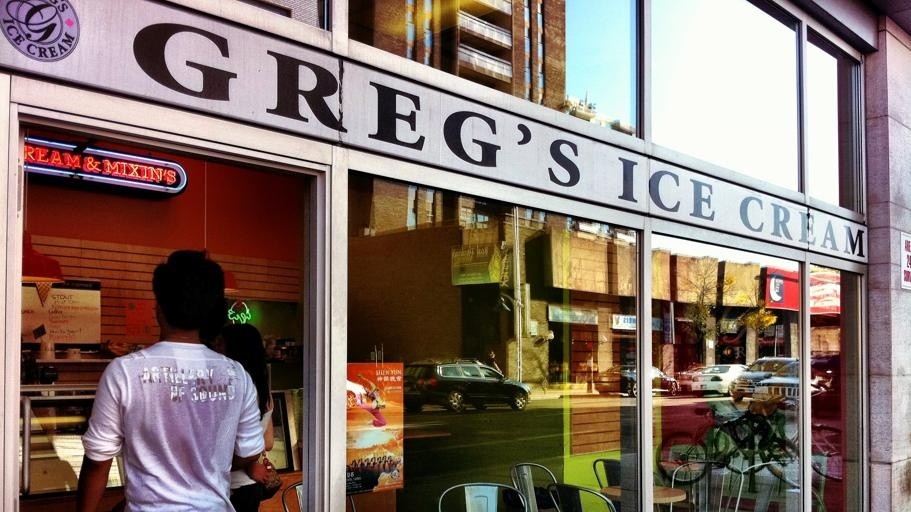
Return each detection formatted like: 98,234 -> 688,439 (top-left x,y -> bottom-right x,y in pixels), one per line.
656,400 -> 843,488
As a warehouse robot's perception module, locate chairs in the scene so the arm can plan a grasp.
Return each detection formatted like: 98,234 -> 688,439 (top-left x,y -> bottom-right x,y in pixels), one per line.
592,458 -> 622,488
670,460 -> 746,512
510,462 -> 556,511
547,483 -> 617,510
440,484 -> 527,512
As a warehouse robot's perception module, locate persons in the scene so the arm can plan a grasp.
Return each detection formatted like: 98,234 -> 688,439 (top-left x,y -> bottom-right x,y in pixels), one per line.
78,250 -> 265,512
487,350 -> 503,374
209,324 -> 274,511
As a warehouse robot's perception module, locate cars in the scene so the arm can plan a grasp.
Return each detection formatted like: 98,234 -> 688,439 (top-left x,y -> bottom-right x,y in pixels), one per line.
404,359 -> 530,413
594,356 -> 842,401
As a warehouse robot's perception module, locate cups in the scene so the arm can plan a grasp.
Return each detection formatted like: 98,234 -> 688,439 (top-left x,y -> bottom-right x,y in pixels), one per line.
41,341 -> 55,359
66,348 -> 80,360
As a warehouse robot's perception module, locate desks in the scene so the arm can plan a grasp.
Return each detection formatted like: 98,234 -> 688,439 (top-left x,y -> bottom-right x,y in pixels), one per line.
601,483 -> 685,503
